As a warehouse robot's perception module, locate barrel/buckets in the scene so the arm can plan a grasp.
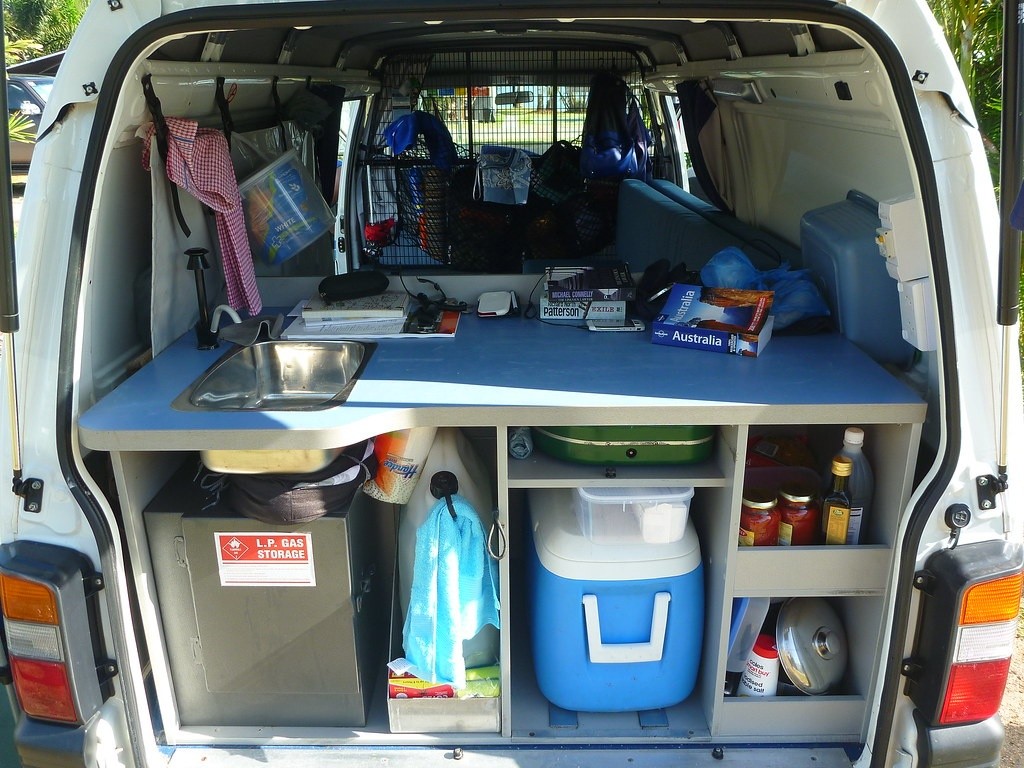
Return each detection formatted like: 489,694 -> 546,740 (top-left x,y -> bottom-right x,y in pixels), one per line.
220,129 -> 336,271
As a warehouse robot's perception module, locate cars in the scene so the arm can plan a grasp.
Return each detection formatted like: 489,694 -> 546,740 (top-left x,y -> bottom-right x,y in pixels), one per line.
6,51 -> 65,176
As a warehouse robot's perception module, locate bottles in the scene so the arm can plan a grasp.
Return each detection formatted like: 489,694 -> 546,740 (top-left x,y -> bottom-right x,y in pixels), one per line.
821,427 -> 875,545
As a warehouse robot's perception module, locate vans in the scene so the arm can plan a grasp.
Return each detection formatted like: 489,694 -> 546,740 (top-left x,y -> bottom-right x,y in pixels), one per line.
0,0 -> 1024,768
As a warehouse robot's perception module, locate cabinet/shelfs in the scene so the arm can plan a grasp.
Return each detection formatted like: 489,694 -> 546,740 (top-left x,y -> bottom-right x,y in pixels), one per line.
79,307 -> 927,745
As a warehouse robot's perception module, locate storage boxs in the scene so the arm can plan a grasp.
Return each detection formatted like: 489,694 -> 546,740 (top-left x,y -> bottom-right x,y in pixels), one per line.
800,188 -> 922,371
575,488 -> 695,543
387,505 -> 502,734
524,488 -> 705,712
726,597 -> 770,672
539,262 -> 635,320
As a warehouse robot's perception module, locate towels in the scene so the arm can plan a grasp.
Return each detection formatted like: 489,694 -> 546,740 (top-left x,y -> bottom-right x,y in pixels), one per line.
402,493 -> 501,691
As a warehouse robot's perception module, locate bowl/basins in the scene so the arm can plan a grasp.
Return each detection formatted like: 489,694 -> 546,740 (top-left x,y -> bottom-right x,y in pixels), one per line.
572,489 -> 695,545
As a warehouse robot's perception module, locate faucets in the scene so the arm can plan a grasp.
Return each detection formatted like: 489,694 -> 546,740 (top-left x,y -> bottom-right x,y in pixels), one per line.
194,304 -> 242,351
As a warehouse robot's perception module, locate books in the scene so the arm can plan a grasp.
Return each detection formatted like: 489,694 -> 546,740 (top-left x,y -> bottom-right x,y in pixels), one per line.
280,291 -> 462,339
546,262 -> 636,303
651,282 -> 775,358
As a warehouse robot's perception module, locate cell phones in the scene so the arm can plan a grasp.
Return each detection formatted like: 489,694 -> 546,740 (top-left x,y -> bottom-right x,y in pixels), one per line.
587,318 -> 646,332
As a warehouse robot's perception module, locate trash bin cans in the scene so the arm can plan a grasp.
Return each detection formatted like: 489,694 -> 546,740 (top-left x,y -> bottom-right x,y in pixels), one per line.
473,96 -> 494,124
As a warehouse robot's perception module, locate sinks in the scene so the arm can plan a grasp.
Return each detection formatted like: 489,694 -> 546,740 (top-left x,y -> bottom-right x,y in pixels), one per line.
169,339 -> 380,475
